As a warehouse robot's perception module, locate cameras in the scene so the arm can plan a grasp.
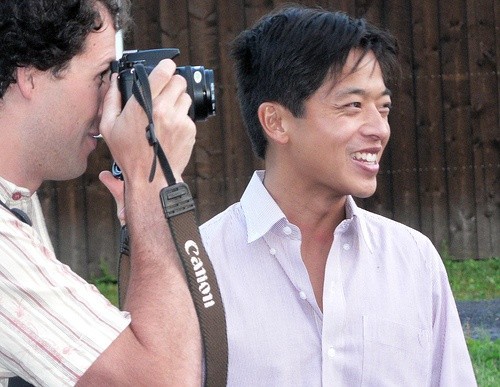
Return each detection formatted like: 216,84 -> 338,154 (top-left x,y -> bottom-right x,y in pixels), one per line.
110,48 -> 217,123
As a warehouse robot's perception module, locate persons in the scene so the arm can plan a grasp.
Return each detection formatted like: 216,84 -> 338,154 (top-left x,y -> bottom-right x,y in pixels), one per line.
1,0 -> 203,387
198,6 -> 477,387
